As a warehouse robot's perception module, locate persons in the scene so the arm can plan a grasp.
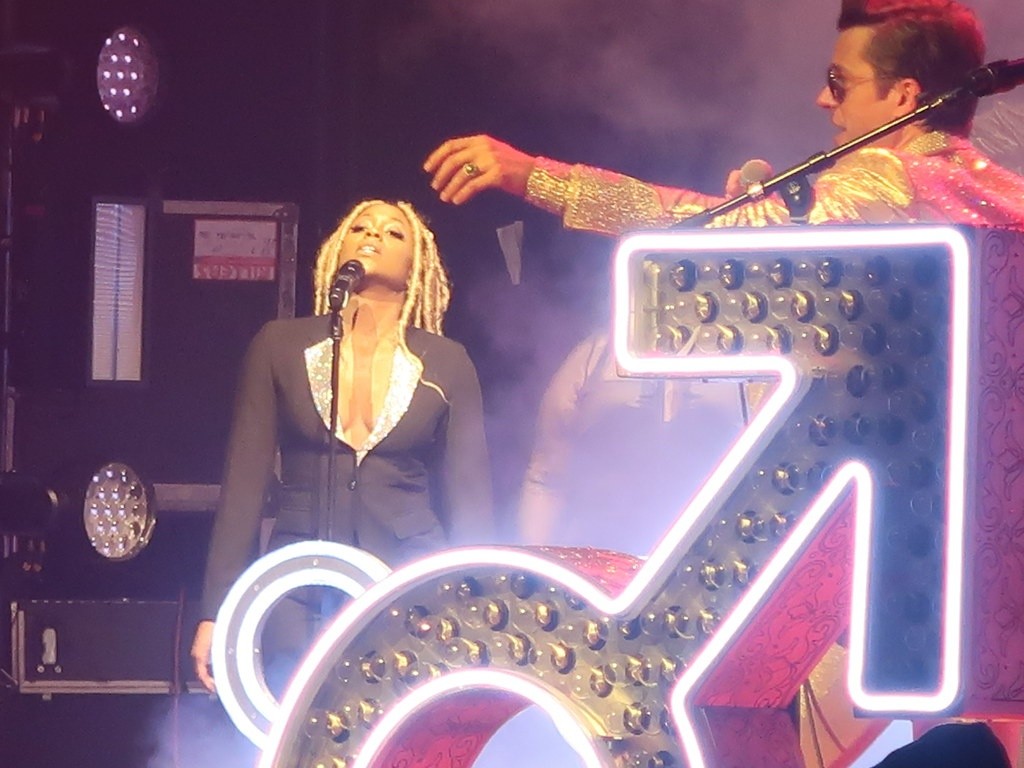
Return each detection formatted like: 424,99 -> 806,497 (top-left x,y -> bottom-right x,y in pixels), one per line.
515,318 -> 770,561
419,0 -> 1024,228
187,196 -> 496,694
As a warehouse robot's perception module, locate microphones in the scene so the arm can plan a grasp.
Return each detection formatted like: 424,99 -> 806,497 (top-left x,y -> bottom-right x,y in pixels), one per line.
326,259 -> 364,309
963,56 -> 1023,98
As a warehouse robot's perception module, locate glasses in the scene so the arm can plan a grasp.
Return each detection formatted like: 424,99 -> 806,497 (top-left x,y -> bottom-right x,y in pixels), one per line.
826,64 -> 895,105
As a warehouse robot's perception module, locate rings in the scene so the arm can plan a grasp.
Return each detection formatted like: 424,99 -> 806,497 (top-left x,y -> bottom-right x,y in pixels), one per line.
462,163 -> 480,177
